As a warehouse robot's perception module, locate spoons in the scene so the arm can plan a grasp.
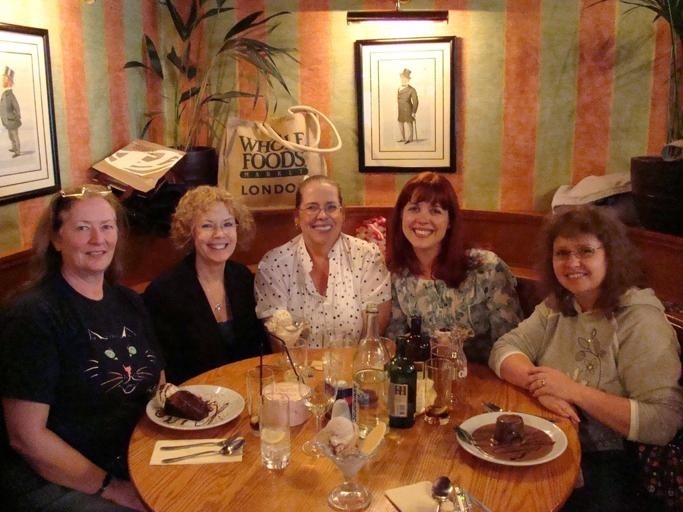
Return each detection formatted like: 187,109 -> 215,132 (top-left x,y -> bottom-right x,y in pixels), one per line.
161,437 -> 250,467
480,399 -> 503,415
428,472 -> 454,512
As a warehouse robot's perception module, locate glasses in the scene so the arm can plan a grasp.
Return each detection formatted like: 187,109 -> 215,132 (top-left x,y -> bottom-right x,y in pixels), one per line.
59,182 -> 114,200
556,245 -> 594,258
295,203 -> 342,218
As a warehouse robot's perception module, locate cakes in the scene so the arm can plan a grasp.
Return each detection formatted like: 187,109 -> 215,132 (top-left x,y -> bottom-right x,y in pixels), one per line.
156,383 -> 209,421
494,414 -> 525,444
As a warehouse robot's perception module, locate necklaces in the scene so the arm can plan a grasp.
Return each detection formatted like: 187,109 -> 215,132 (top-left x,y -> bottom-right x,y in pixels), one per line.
199,276 -> 227,313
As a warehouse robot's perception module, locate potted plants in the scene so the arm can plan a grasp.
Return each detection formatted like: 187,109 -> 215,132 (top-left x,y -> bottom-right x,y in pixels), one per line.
123,0 -> 301,211
586,0 -> 682,237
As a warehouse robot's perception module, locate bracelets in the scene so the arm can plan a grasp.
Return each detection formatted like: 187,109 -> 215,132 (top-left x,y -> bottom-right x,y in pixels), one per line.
87,469 -> 113,497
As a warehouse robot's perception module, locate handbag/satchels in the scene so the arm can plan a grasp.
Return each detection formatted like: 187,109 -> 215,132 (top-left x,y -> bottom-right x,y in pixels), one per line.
217,109 -> 329,216
92,138 -> 187,198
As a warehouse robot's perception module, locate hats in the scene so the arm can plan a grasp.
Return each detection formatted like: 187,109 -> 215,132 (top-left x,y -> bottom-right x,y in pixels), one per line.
401,68 -> 412,78
3,68 -> 15,81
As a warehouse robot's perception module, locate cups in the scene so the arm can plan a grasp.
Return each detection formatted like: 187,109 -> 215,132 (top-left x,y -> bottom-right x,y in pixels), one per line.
245,309 -> 385,511
422,321 -> 467,423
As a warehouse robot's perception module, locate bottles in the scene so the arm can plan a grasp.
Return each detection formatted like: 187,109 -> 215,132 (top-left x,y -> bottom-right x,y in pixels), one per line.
383,335 -> 419,430
350,301 -> 392,439
405,311 -> 431,373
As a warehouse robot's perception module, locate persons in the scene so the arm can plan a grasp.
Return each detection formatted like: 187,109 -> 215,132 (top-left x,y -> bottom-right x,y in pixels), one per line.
386,169 -> 522,368
0,67 -> 23,160
139,183 -> 266,377
487,205 -> 682,512
0,185 -> 167,512
251,174 -> 391,350
396,67 -> 418,145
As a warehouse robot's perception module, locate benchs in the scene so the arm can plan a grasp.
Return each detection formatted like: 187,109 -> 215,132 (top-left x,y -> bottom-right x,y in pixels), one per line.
130,255 -> 681,340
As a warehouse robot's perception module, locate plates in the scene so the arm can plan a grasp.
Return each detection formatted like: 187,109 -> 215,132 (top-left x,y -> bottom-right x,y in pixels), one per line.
454,411 -> 567,467
142,385 -> 245,429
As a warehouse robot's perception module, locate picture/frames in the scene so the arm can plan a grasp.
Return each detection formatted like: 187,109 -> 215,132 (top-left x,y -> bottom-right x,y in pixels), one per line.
0,21 -> 61,207
354,36 -> 457,174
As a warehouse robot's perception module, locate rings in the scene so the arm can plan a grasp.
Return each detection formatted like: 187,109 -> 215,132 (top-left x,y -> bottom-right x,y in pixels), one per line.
541,379 -> 545,386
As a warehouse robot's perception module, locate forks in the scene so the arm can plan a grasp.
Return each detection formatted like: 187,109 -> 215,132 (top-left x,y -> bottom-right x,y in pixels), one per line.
160,429 -> 241,452
450,423 -> 555,448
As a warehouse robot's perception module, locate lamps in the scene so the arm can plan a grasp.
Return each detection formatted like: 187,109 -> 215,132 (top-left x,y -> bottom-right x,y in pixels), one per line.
347,1 -> 451,26
